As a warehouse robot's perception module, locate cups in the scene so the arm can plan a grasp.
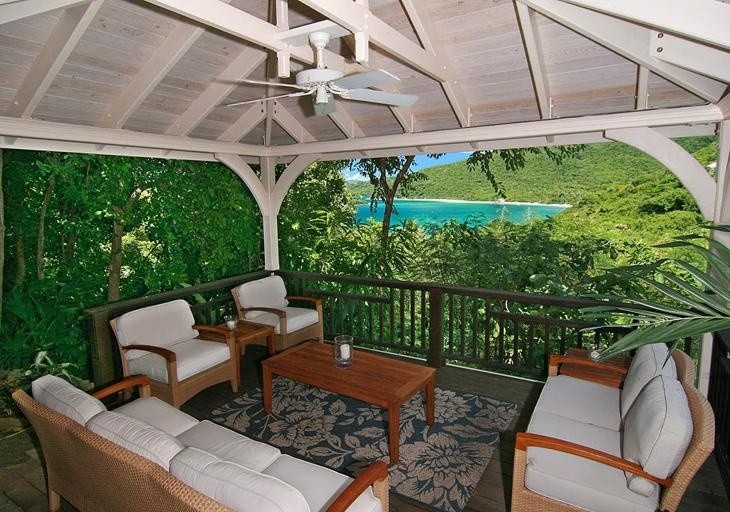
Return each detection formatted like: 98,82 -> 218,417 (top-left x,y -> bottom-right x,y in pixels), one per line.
334,335 -> 354,369
224,314 -> 238,331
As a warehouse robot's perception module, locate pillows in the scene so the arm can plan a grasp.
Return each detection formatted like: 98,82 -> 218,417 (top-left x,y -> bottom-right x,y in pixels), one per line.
115,299 -> 200,361
623,375 -> 694,497
86,411 -> 185,473
236,275 -> 289,319
620,342 -> 678,426
170,447 -> 311,512
31,373 -> 108,428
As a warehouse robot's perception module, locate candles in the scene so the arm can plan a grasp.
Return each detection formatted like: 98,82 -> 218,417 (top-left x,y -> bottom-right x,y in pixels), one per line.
340,344 -> 351,360
227,321 -> 236,329
591,351 -> 600,359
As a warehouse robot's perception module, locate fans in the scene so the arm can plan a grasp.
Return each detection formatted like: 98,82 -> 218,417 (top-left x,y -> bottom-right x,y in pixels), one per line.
214,32 -> 418,117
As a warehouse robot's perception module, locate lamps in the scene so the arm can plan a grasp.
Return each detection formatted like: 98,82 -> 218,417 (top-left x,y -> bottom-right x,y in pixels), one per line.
316,86 -> 328,104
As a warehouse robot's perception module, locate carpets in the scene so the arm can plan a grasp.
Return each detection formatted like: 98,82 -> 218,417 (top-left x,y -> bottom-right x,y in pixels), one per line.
205,373 -> 521,512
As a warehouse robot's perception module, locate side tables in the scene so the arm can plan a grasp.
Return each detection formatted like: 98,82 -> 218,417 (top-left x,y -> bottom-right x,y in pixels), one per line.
559,346 -> 632,391
200,319 -> 276,386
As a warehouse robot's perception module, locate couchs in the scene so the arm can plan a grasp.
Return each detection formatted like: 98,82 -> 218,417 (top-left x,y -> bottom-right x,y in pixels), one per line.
510,348 -> 715,512
12,374 -> 390,512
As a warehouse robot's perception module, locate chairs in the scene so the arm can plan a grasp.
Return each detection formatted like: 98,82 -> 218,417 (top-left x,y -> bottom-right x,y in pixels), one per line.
230,275 -> 324,356
109,298 -> 238,410
